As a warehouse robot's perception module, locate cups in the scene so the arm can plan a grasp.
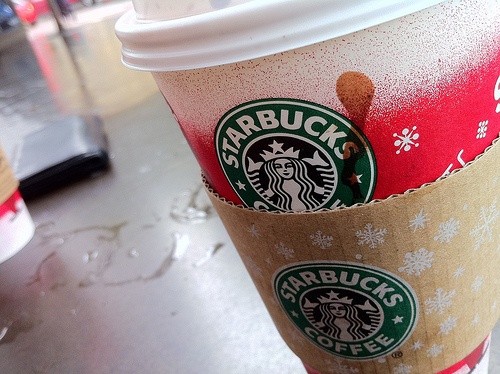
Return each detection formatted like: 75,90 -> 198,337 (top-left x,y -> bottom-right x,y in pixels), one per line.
114,0 -> 499,374
